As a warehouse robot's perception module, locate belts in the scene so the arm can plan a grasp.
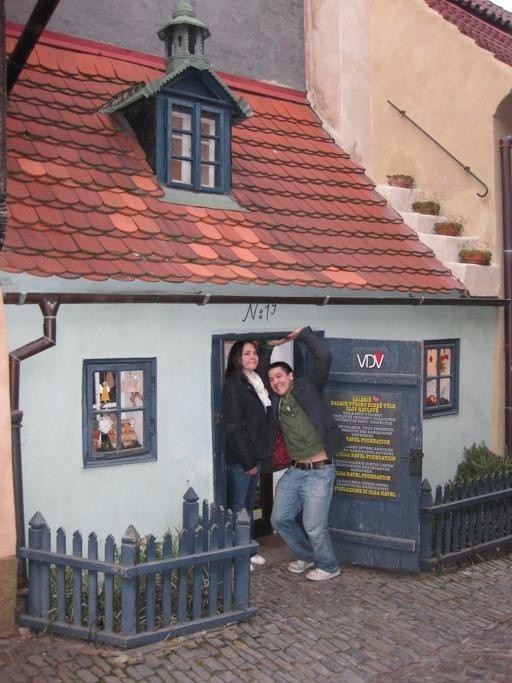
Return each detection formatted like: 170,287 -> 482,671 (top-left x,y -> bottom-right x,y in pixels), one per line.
291,459 -> 332,470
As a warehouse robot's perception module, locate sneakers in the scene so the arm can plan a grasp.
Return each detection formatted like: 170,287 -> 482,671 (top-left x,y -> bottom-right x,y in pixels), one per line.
250,554 -> 266,573
287,559 -> 314,574
305,567 -> 340,581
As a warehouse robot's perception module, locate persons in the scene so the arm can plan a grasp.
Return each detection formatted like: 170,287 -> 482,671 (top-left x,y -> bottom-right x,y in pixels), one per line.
223,341 -> 276,575
256,325 -> 347,580
